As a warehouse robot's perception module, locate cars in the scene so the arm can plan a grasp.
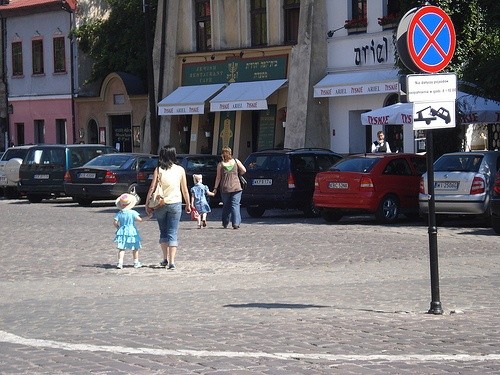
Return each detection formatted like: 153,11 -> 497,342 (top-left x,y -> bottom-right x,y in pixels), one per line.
63,152 -> 155,207
137,153 -> 223,208
313,152 -> 426,225
418,150 -> 500,231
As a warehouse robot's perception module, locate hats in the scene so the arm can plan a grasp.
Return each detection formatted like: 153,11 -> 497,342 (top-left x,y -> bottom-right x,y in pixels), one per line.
115,194 -> 138,210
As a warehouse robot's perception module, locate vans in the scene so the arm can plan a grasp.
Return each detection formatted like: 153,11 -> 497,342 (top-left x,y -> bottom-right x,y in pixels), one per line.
18,144 -> 121,203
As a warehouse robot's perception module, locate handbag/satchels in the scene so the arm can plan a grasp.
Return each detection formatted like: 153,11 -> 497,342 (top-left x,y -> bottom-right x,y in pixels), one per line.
191,209 -> 199,220
239,175 -> 247,189
148,184 -> 164,209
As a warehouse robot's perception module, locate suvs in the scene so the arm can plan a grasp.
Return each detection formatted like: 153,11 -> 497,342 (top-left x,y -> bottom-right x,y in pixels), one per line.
0,145 -> 38,199
238,146 -> 346,219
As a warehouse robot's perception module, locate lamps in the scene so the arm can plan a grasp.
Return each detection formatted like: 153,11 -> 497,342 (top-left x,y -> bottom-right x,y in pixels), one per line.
328,25 -> 347,37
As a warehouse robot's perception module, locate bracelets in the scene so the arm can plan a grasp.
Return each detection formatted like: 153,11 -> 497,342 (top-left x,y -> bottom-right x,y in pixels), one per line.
214,187 -> 218,190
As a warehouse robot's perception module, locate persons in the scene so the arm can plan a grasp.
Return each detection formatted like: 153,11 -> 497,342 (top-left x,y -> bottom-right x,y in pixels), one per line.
213,145 -> 246,229
113,193 -> 144,268
146,144 -> 191,269
191,174 -> 215,228
371,131 -> 392,154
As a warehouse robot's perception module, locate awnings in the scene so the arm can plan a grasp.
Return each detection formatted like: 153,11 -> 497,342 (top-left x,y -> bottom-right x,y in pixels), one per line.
313,69 -> 406,98
210,79 -> 288,111
157,83 -> 228,115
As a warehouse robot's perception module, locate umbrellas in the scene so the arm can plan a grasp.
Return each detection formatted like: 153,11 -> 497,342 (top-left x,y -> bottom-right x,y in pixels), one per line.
361,91 -> 500,123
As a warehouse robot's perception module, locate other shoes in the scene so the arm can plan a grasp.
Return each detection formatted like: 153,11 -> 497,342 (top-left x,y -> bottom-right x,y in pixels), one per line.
169,264 -> 175,269
198,225 -> 201,229
161,259 -> 168,266
203,219 -> 206,227
134,262 -> 142,268
117,263 -> 123,269
